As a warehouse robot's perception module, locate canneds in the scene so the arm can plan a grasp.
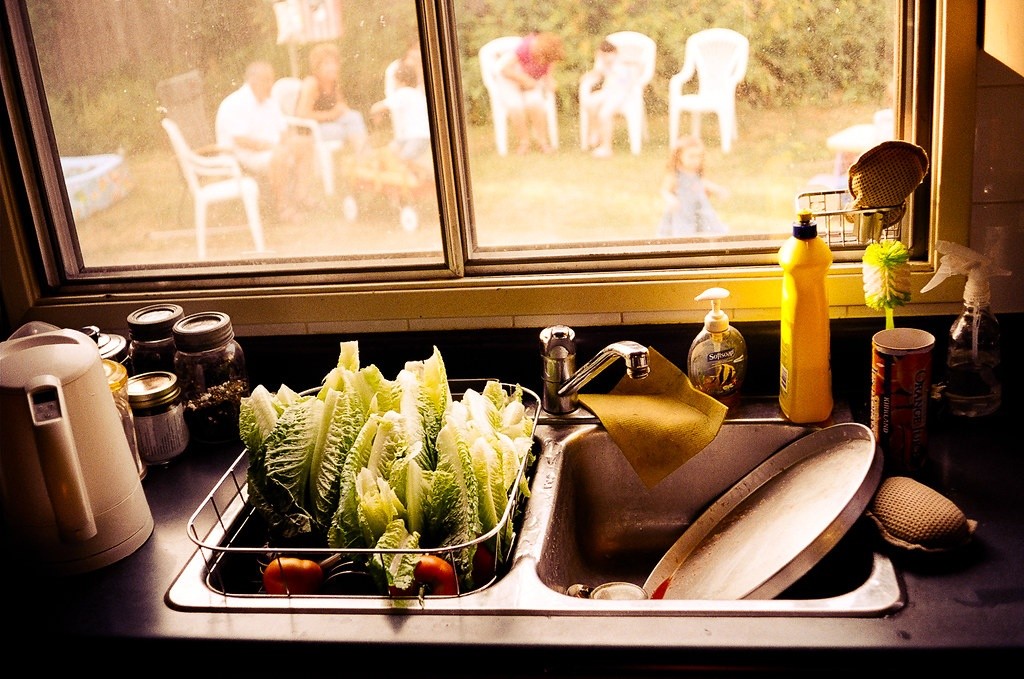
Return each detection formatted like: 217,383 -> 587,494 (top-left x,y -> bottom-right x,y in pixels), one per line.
125,370 -> 190,462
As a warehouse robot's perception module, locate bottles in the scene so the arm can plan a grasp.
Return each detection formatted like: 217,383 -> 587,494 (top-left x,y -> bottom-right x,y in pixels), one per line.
776,207 -> 834,426
77,302 -> 251,487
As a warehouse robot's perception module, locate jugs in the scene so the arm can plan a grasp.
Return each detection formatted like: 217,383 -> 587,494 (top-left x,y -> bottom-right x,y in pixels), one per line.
0,321 -> 154,577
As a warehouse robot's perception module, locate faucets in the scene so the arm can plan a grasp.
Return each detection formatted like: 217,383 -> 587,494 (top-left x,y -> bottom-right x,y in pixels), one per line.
539,322 -> 651,414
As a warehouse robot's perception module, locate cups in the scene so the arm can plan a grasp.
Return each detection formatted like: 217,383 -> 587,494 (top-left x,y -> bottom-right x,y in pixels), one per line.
566,581 -> 649,600
870,328 -> 936,472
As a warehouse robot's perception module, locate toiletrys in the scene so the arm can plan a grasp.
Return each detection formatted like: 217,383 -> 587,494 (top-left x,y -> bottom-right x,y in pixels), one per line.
921,240 -> 1013,417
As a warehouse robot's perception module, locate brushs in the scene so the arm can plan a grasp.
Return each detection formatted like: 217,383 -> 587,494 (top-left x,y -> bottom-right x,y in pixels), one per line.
861,240 -> 912,332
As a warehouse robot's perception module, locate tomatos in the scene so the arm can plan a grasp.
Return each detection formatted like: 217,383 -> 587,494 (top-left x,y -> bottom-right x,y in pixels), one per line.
263,556 -> 324,594
389,556 -> 460,597
474,535 -> 493,572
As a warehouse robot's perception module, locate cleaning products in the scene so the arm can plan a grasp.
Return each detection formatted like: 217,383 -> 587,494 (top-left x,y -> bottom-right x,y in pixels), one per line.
685,287 -> 748,400
776,210 -> 834,425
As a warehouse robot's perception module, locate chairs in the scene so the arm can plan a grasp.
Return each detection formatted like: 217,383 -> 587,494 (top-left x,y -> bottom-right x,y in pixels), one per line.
385,59 -> 431,139
270,76 -> 368,193
154,68 -> 229,219
578,30 -> 656,155
160,118 -> 266,261
668,28 -> 749,153
479,37 -> 560,156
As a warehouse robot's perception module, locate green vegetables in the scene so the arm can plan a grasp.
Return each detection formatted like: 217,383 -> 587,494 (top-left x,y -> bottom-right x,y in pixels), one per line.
239,341 -> 537,593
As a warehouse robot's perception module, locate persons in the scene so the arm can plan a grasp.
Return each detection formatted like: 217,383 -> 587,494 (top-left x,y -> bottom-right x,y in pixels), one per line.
578,38 -> 636,159
295,40 -> 368,163
498,29 -> 566,157
660,134 -> 730,239
213,60 -> 316,181
370,40 -> 430,160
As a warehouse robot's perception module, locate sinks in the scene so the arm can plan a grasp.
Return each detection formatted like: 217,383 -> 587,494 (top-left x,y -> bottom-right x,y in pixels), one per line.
536,396 -> 901,618
164,404 -> 545,613
